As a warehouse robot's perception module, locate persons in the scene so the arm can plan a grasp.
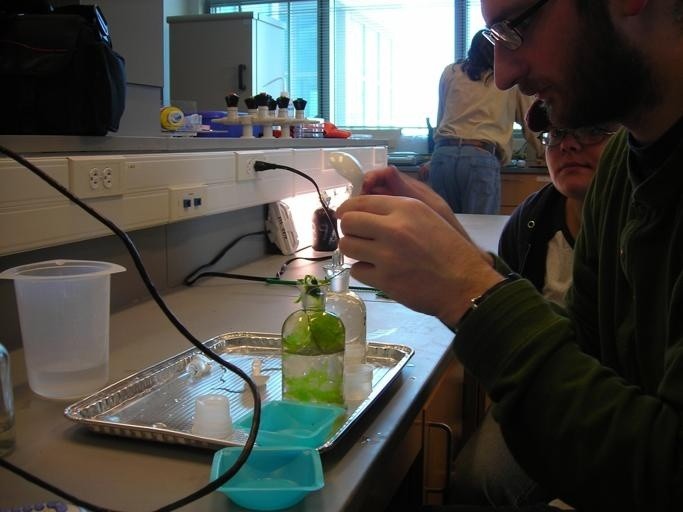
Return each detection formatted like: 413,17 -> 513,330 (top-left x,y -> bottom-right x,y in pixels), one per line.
469,95 -> 620,510
417,30 -> 544,214
329,0 -> 683,512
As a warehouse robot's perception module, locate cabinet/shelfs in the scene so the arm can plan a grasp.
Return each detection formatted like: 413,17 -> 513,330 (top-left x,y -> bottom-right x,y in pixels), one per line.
501,173 -> 584,239
167,11 -> 284,114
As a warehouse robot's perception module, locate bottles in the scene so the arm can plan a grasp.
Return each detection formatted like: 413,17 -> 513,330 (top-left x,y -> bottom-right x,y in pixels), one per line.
318,249 -> 366,368
282,282 -> 345,412
159,105 -> 183,132
0,344 -> 15,434
309,196 -> 339,253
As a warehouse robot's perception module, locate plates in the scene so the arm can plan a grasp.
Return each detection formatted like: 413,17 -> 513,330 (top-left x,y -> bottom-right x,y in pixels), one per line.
210,443 -> 325,511
232,402 -> 345,448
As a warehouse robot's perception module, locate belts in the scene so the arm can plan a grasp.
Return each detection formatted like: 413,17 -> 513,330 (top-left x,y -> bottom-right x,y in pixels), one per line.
436,138 -> 501,157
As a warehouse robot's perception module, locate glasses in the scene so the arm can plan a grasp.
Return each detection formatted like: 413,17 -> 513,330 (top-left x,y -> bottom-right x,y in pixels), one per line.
482,0 -> 550,50
537,127 -> 614,146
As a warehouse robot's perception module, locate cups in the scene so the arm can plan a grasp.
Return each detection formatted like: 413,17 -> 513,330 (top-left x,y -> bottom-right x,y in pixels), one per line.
340,364 -> 373,401
189,394 -> 235,440
1,258 -> 127,403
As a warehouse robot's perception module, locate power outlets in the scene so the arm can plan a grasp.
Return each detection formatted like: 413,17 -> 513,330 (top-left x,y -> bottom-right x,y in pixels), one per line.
68,156 -> 126,201
236,152 -> 265,182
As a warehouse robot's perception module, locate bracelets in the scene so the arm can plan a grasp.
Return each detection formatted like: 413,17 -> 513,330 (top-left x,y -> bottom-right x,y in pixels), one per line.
452,273 -> 516,331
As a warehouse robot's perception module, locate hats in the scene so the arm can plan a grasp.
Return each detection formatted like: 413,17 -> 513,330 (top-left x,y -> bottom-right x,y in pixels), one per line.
525,97 -> 551,133
